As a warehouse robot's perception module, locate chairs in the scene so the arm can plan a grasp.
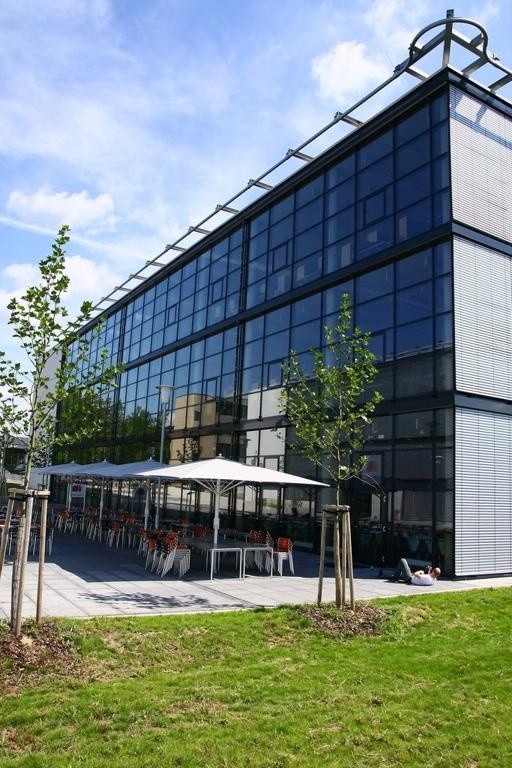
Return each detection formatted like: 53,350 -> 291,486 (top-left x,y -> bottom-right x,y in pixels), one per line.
1,509 -> 296,580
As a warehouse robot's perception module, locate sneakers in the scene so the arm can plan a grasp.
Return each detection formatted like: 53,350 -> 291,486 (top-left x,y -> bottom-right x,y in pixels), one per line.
378,570 -> 383,579
390,576 -> 399,580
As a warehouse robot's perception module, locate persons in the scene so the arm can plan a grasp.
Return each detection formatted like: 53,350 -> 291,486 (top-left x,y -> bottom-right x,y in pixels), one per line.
388,558 -> 441,586
